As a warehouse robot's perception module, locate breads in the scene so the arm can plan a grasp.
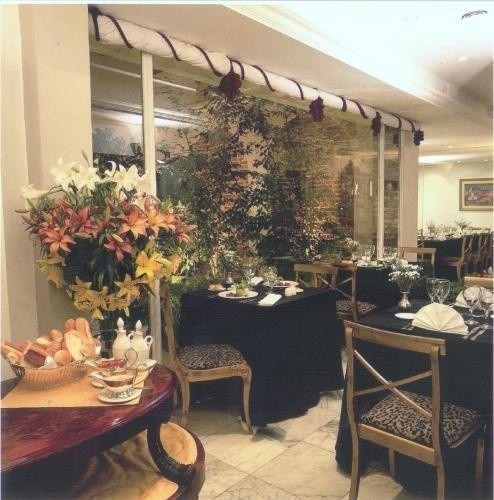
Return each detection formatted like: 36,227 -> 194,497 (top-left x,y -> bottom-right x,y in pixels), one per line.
25,345 -> 52,366
65,319 -> 75,331
1,342 -> 24,365
76,319 -> 98,358
63,331 -> 84,363
51,330 -> 63,345
36,335 -> 50,347
55,350 -> 71,366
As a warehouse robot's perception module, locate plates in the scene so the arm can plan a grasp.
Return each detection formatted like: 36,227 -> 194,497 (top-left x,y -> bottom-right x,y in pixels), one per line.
92,357 -> 157,371
89,370 -> 149,388
206,288 -> 225,291
261,281 -> 299,287
97,385 -> 142,403
394,313 -> 415,320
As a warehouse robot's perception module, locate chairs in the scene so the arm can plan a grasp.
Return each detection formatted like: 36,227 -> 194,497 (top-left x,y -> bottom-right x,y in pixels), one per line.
294,265 -> 379,335
160,289 -> 254,437
462,277 -> 494,306
441,234 -> 475,285
396,244 -> 436,274
470,233 -> 490,278
344,318 -> 489,499
485,231 -> 494,274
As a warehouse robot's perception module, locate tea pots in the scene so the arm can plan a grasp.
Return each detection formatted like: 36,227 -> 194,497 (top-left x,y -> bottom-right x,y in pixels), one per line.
111,317 -> 133,360
129,320 -> 153,365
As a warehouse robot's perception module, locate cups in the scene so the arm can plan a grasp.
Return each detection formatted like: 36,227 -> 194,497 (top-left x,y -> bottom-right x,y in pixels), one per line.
217,291 -> 258,300
434,279 -> 450,304
82,347 -> 137,376
462,283 -> 481,325
425,278 -> 438,304
477,286 -> 493,329
87,362 -> 149,400
360,243 -> 375,264
382,247 -> 400,265
241,257 -> 256,290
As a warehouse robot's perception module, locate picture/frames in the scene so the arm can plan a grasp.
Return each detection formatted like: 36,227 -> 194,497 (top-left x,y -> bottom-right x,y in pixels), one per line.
459,179 -> 494,211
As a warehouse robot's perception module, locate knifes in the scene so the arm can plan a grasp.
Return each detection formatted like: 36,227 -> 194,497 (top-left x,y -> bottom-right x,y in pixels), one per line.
470,328 -> 488,341
461,326 -> 480,341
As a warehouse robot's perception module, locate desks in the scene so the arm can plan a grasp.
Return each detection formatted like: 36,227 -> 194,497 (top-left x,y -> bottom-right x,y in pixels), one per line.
0,355 -> 206,500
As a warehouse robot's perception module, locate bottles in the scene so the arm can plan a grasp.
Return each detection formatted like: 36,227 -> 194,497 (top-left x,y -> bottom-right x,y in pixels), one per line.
224,272 -> 234,288
